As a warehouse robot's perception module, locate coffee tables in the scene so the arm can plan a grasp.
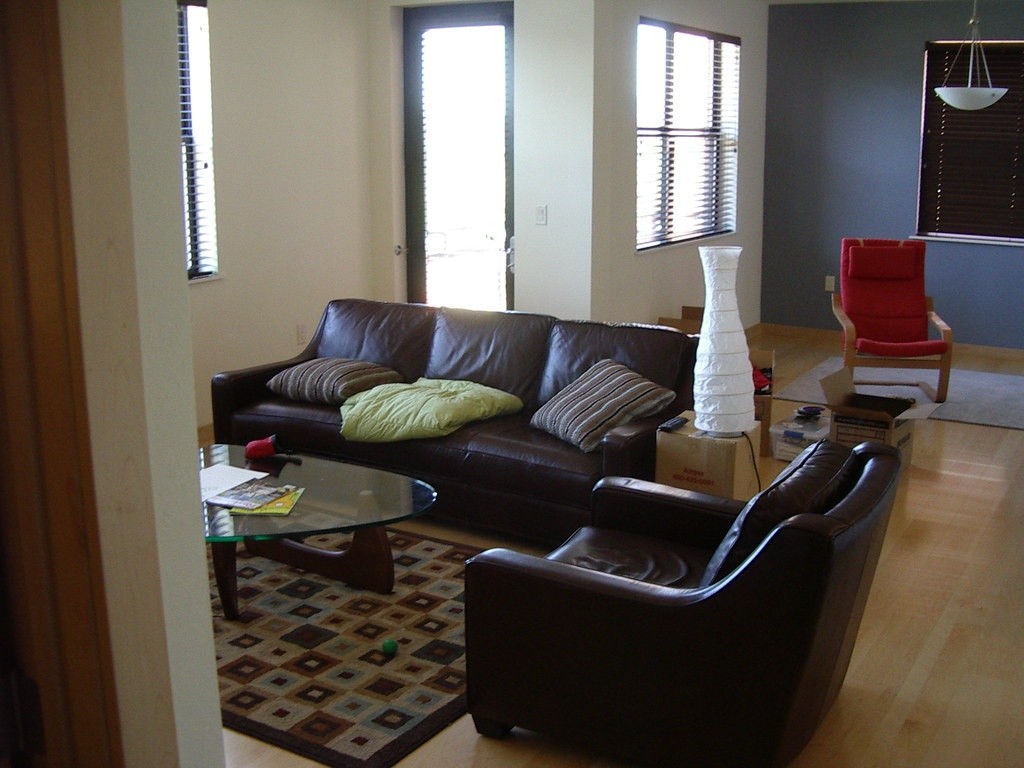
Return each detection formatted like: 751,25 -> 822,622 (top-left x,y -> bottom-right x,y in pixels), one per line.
198,444 -> 436,618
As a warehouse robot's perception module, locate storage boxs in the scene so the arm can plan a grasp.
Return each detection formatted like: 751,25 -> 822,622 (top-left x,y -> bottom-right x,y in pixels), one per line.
658,304 -> 705,334
656,411 -> 762,500
748,346 -> 776,456
818,367 -> 943,470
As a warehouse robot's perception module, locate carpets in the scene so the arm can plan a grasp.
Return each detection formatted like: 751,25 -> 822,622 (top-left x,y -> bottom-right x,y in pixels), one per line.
773,357 -> 1024,430
206,502 -> 485,768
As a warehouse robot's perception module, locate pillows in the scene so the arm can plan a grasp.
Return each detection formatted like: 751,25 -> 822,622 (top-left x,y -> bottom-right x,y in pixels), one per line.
265,357 -> 398,404
531,359 -> 676,452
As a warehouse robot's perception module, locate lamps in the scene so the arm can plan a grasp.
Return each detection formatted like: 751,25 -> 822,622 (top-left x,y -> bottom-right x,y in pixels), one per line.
934,0 -> 1009,111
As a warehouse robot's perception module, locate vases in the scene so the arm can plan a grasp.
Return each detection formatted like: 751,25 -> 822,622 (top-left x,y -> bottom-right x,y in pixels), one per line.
694,245 -> 756,438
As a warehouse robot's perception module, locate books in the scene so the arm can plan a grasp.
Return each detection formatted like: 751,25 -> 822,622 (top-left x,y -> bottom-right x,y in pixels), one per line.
207,478 -> 305,516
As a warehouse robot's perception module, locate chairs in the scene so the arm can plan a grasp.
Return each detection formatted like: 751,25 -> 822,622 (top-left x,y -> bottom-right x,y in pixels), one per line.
832,238 -> 953,404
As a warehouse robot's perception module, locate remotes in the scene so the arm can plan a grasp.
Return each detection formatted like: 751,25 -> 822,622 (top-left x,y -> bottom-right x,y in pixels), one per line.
659,417 -> 689,433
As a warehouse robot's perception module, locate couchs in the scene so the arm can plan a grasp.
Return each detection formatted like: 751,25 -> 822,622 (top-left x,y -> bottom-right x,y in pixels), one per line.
208,296 -> 701,547
463,440 -> 903,768
